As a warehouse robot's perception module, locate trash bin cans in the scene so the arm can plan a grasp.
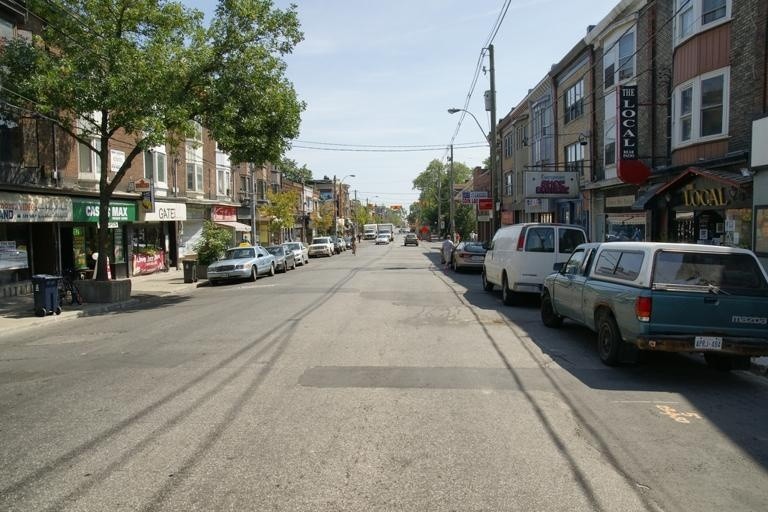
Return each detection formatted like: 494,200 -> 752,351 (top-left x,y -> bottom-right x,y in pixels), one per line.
182,259 -> 197,283
32,274 -> 63,317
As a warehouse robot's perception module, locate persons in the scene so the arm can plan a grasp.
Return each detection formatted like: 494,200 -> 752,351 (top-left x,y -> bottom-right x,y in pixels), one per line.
456,232 -> 460,244
358,233 -> 361,242
442,235 -> 456,275
351,235 -> 356,242
469,230 -> 476,241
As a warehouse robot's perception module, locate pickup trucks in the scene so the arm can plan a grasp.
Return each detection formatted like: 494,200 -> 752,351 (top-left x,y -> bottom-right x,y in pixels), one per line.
538,239 -> 767,372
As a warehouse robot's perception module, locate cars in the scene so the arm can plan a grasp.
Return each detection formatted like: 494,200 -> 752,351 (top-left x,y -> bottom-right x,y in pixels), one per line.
308,236 -> 335,258
206,245 -> 277,287
404,233 -> 418,246
440,241 -> 487,272
375,234 -> 389,245
265,241 -> 309,273
332,236 -> 356,254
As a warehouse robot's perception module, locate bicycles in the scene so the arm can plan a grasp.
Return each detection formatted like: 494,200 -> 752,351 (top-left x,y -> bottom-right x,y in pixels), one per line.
54,265 -> 83,307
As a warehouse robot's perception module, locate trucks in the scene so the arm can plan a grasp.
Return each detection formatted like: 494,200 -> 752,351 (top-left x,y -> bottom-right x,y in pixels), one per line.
378,223 -> 394,242
363,224 -> 378,239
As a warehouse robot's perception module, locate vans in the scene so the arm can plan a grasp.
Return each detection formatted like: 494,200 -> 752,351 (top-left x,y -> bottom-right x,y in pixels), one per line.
480,222 -> 589,306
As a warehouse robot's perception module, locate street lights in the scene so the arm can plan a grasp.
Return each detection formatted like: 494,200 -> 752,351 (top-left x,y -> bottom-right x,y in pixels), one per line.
448,108 -> 497,238
339,174 -> 355,219
